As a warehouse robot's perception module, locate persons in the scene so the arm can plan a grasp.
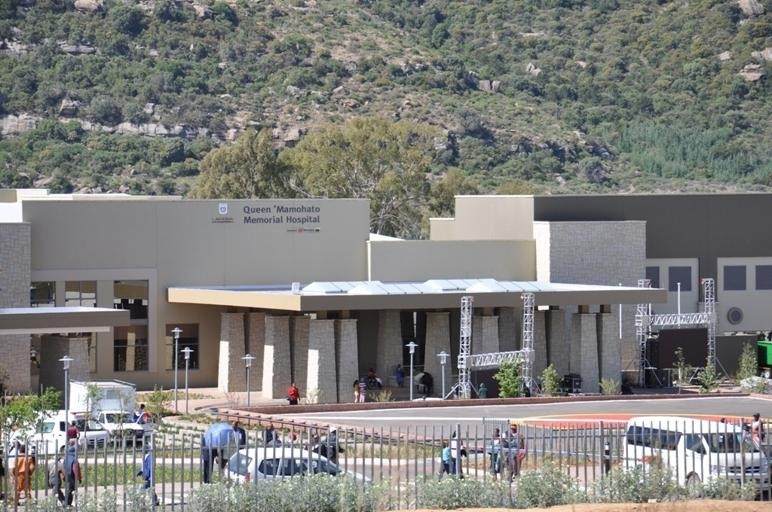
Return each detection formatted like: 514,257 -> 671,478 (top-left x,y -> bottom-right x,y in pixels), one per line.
353,380 -> 360,403
479,382 -> 487,398
366,369 -> 381,390
231,419 -> 247,453
359,377 -> 367,403
135,403 -> 146,423
61,446 -> 83,507
449,431 -> 466,482
751,411 -> 764,447
47,454 -> 65,508
488,422 -> 527,481
437,440 -> 455,482
67,420 -> 80,458
262,421 -> 278,445
287,382 -> 301,405
132,444 -> 160,507
396,363 -> 404,388
200,445 -> 221,485
0,448 -> 8,499
11,444 -> 36,506
290,429 -> 347,466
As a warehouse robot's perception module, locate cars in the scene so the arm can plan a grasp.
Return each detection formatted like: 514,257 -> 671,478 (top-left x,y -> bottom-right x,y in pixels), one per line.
2,409 -> 154,454
223,447 -> 374,488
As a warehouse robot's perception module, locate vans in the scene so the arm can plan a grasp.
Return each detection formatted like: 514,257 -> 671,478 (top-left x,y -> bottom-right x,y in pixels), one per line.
622,415 -> 771,498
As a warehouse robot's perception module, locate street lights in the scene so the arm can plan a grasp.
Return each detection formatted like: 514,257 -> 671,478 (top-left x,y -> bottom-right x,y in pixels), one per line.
59,355 -> 75,442
437,350 -> 451,400
241,353 -> 257,408
170,327 -> 183,414
180,346 -> 194,415
405,341 -> 419,401
